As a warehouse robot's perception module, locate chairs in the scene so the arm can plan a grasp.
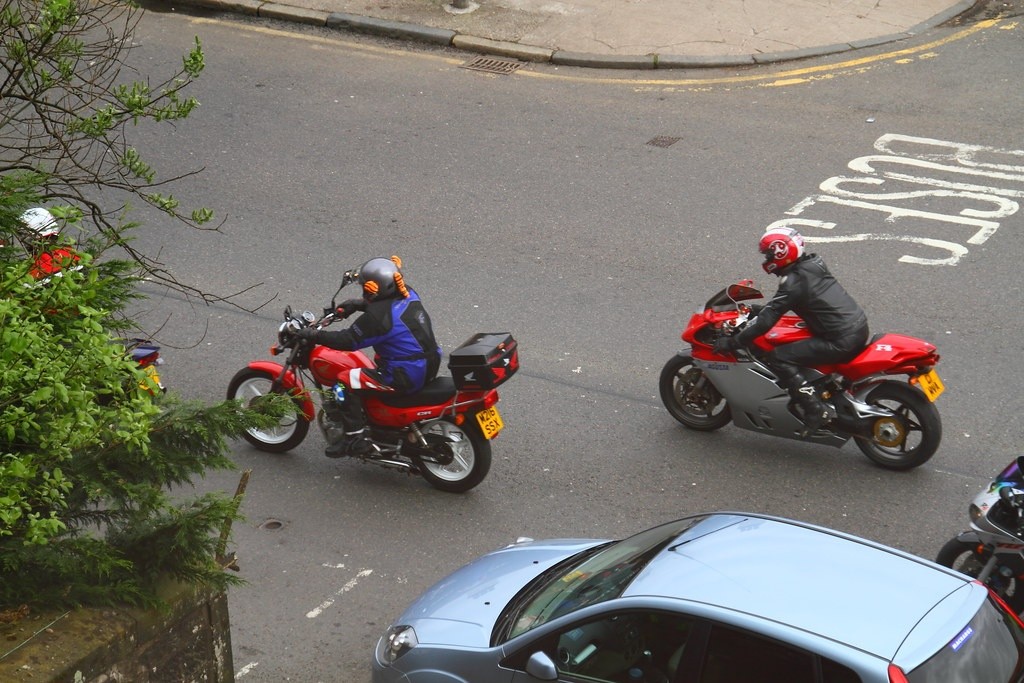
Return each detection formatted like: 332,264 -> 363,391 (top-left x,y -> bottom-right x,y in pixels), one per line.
667,642 -> 720,682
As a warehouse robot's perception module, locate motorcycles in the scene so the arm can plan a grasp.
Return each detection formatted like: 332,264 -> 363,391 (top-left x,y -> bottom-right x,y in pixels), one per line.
84,338 -> 166,411
658,279 -> 945,469
227,269 -> 519,493
935,456 -> 1024,616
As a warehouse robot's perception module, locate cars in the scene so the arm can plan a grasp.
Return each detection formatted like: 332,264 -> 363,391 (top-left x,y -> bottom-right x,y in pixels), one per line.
371,511 -> 1024,683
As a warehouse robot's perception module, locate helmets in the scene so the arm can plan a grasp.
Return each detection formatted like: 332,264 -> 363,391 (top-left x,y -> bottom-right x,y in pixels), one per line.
359,255 -> 406,302
15,207 -> 60,253
758,226 -> 805,274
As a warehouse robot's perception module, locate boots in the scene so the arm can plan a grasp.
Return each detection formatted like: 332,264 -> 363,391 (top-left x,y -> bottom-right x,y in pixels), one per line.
785,373 -> 835,439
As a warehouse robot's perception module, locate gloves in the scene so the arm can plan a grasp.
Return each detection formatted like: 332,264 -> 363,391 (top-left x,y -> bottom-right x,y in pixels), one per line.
336,299 -> 356,319
712,337 -> 738,355
295,328 -> 318,346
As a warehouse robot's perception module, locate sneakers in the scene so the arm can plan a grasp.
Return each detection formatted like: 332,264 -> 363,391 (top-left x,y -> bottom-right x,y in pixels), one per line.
325,426 -> 374,458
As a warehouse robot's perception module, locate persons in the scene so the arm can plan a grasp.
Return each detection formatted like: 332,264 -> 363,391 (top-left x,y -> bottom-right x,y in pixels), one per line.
292,256 -> 442,458
15,207 -> 82,317
712,227 -> 869,439
